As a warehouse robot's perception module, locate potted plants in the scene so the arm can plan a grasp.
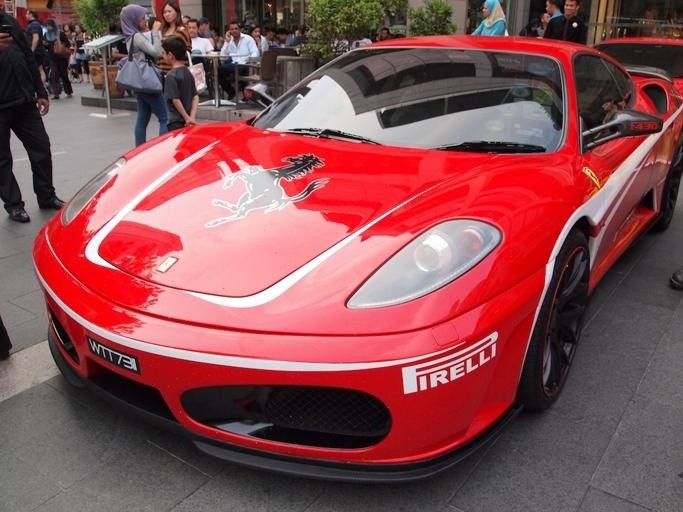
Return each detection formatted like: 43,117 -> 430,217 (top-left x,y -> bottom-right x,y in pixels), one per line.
70,1 -> 131,100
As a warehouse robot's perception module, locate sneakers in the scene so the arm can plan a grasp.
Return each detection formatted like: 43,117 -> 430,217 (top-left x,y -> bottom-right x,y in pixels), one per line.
227,89 -> 236,99
51,96 -> 58,100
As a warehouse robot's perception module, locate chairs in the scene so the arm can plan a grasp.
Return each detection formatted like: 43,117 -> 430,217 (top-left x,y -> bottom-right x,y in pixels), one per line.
238,51 -> 279,108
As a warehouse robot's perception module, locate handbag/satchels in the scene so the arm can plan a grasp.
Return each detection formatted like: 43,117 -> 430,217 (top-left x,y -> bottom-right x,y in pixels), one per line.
116,56 -> 164,94
53,41 -> 71,56
185,51 -> 207,94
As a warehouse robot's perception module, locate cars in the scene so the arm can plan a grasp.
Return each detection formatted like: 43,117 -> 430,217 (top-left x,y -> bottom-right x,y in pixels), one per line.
592,35 -> 682,104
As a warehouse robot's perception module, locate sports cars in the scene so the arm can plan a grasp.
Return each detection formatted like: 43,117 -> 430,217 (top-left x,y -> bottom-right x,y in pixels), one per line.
30,34 -> 683,482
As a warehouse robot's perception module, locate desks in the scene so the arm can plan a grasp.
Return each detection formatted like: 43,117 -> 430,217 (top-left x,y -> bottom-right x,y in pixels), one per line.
189,52 -> 247,109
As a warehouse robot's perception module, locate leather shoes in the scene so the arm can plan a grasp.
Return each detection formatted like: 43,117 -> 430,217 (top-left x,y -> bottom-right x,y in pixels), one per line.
7,206 -> 30,222
39,199 -> 65,210
670,268 -> 683,290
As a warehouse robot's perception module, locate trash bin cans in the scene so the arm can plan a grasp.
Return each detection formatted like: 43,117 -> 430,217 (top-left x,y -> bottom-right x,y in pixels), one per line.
274,55 -> 314,97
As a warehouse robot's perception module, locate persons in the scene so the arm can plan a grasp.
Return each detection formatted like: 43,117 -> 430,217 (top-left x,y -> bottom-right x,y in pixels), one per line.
518,1 -> 586,46
466,0 -> 506,36
118,4 -> 167,148
154,3 -> 191,76
159,33 -> 196,131
597,97 -> 624,127
0,9 -> 397,101
0,8 -> 68,225
